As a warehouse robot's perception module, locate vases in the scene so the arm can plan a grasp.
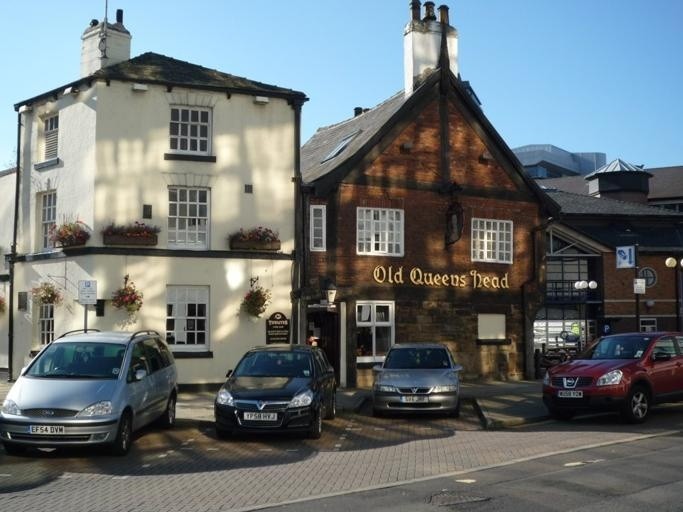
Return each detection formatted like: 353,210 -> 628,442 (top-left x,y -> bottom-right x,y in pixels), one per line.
103,235 -> 157,245
229,239 -> 281,250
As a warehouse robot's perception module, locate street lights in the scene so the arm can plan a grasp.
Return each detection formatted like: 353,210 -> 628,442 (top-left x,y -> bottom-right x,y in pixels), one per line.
575,281 -> 597,345
665,258 -> 683,332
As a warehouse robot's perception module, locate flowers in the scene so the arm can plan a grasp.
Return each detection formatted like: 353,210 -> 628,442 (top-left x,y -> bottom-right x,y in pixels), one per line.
104,223 -> 158,239
242,287 -> 272,319
46,222 -> 90,246
28,285 -> 64,309
111,284 -> 143,314
228,227 -> 281,242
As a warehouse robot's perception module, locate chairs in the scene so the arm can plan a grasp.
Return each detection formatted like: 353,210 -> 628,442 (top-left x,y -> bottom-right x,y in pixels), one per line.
428,351 -> 446,367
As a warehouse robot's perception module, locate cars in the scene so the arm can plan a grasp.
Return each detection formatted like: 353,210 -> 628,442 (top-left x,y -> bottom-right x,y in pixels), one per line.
0,329 -> 179,455
371,342 -> 464,418
213,343 -> 338,439
543,331 -> 683,424
